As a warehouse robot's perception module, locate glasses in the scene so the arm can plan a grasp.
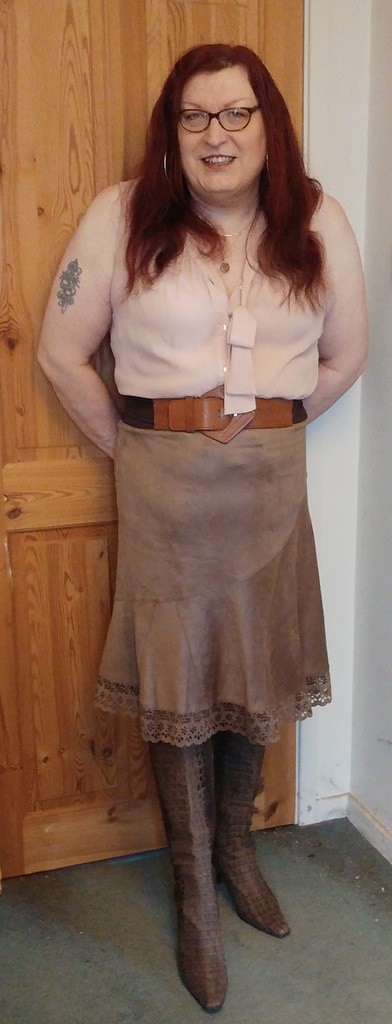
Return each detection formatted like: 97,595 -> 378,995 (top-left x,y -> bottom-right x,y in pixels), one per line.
177,103 -> 261,134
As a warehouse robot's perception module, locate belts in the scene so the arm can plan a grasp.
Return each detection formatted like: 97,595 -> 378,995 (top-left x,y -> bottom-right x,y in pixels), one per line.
121,396 -> 308,444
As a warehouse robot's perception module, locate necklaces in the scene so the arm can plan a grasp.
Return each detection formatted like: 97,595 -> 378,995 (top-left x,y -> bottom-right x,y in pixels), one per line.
216,223 -> 250,273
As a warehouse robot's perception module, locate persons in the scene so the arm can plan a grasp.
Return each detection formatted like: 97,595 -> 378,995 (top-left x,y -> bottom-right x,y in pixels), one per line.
38,45 -> 370,1011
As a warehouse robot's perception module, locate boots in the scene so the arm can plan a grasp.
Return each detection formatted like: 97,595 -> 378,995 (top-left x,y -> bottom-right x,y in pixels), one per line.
147,735 -> 227,1012
211,729 -> 290,938
